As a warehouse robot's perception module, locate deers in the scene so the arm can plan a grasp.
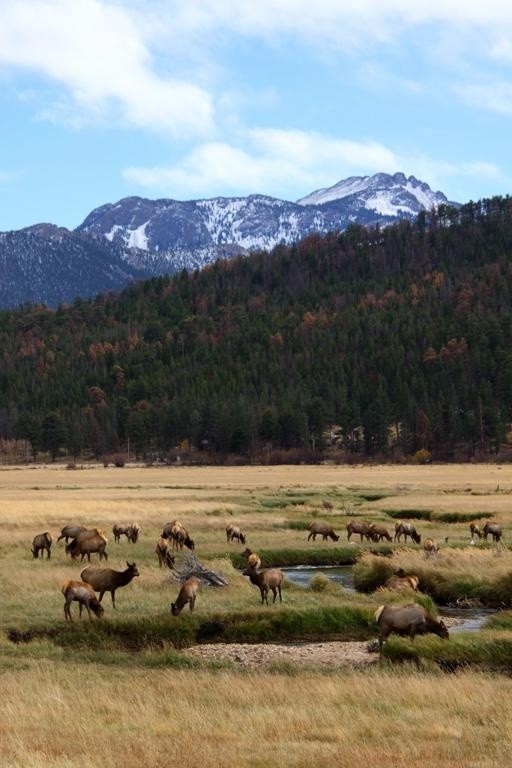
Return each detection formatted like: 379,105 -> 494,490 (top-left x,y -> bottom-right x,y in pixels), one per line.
242,561 -> 284,607
225,524 -> 246,545
307,519 -> 422,545
61,579 -> 105,624
30,519 -> 139,562
424,537 -> 440,554
469,520 -> 503,543
80,560 -> 140,608
156,519 -> 195,570
374,602 -> 449,658
384,567 -> 419,591
171,575 -> 198,617
